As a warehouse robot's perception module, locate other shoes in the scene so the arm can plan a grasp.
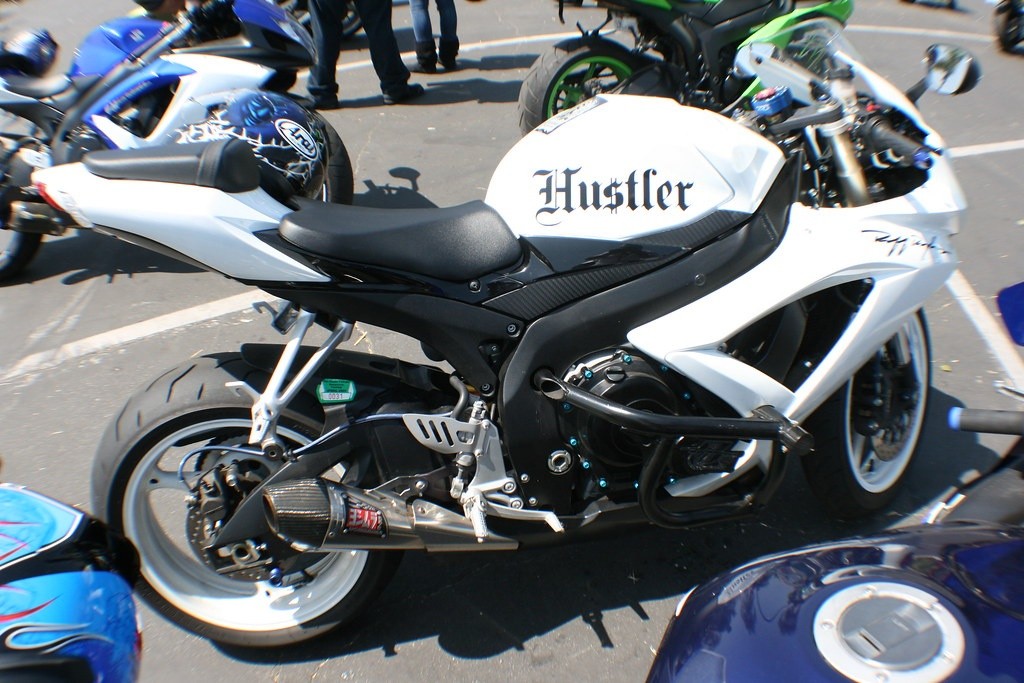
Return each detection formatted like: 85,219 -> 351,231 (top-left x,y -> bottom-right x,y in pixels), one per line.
405,63 -> 437,73
306,93 -> 338,109
383,84 -> 425,104
437,52 -> 464,71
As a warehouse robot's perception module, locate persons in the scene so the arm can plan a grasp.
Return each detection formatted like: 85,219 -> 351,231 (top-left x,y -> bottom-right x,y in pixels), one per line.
305,0 -> 425,111
406,0 -> 463,74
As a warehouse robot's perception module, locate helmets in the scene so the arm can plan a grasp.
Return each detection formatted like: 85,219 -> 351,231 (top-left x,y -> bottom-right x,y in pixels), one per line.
0,483 -> 143,683
174,89 -> 329,200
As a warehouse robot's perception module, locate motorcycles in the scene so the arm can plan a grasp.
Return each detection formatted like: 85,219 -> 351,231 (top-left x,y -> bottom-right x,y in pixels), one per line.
0,0 -> 1024,683
